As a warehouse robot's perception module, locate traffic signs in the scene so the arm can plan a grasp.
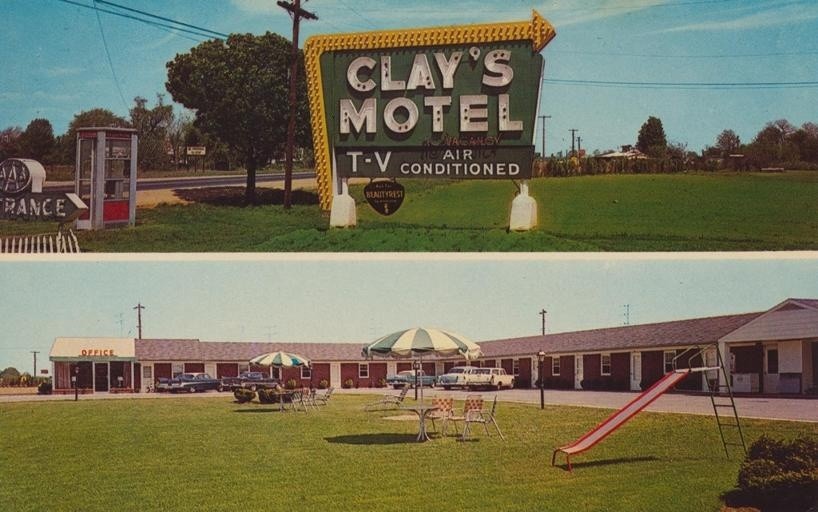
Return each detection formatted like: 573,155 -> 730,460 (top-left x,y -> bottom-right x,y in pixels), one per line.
0,157 -> 90,223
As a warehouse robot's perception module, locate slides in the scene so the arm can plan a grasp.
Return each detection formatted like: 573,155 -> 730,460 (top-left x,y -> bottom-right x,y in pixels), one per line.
552,371 -> 686,473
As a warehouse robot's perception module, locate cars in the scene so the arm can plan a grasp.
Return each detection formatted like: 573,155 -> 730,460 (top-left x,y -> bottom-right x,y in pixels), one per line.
435,365 -> 516,391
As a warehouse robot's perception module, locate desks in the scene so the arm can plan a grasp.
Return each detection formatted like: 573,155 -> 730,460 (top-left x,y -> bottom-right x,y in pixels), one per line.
400,406 -> 442,442
272,392 -> 290,412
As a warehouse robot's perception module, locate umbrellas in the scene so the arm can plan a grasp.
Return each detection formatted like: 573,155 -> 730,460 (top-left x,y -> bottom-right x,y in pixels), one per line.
360,323 -> 482,401
249,347 -> 313,381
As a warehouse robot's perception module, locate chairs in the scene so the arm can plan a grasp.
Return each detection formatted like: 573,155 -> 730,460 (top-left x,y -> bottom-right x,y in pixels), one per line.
363,383 -> 411,411
423,394 -> 507,445
286,385 -> 334,413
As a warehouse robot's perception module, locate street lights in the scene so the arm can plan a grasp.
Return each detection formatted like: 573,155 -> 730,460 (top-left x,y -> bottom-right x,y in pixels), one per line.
74,366 -> 80,401
537,348 -> 546,410
413,360 -> 419,401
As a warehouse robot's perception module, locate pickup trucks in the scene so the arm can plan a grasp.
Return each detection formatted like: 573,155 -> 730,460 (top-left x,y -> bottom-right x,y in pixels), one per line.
387,370 -> 436,388
221,371 -> 280,393
156,371 -> 222,393
